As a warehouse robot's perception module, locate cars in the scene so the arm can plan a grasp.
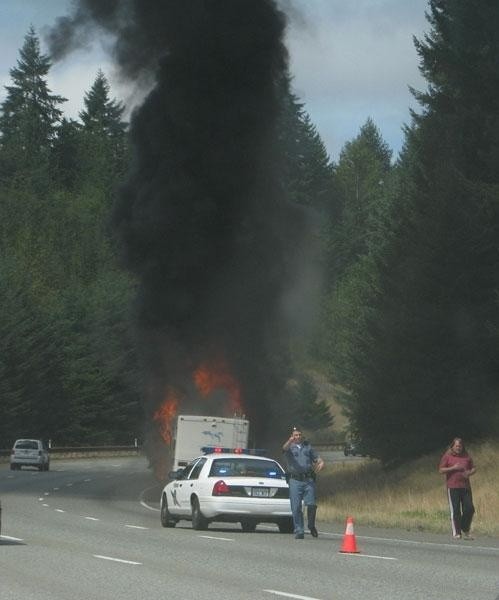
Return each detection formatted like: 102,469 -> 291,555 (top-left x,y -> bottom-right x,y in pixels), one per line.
344,440 -> 366,457
9,437 -> 51,471
159,447 -> 304,532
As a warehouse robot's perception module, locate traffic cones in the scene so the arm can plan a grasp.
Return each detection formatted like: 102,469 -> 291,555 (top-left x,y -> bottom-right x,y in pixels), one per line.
338,516 -> 360,554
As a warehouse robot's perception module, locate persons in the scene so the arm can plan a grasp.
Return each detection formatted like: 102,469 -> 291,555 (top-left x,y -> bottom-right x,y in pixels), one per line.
282,425 -> 324,540
438,436 -> 478,541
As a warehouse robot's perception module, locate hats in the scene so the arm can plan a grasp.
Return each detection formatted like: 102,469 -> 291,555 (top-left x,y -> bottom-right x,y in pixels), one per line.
292,426 -> 302,432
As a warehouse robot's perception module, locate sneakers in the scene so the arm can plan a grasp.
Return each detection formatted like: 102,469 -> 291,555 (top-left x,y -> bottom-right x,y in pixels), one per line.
310,528 -> 318,537
295,534 -> 304,539
465,532 -> 472,537
453,534 -> 461,539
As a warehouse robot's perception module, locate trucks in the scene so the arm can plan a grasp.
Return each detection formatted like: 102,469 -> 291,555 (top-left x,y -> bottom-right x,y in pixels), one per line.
169,414 -> 251,472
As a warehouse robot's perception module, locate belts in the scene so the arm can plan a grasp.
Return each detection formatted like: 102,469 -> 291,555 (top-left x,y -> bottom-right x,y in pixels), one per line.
291,473 -> 313,479
